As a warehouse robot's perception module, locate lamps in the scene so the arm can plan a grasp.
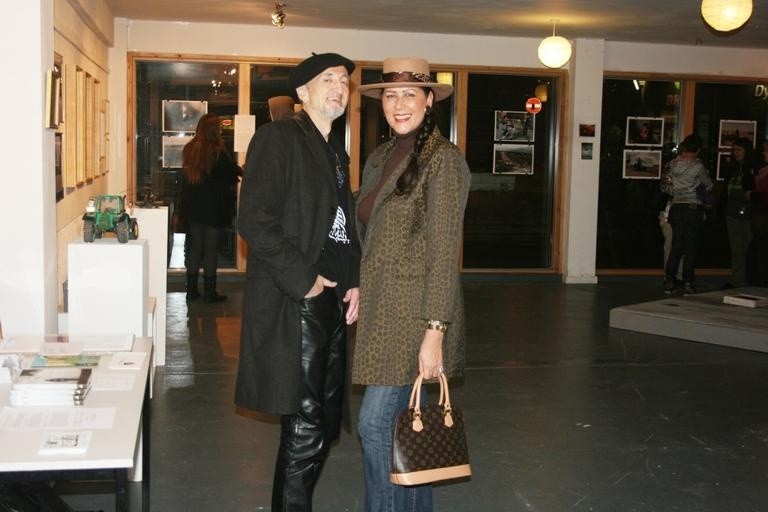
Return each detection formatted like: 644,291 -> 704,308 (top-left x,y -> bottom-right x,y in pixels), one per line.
537,17 -> 573,69
271,1 -> 288,28
701,0 -> 755,32
535,83 -> 549,102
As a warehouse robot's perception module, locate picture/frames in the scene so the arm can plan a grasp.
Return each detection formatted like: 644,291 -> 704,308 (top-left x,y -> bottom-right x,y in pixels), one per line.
98,98 -> 111,175
44,66 -> 61,128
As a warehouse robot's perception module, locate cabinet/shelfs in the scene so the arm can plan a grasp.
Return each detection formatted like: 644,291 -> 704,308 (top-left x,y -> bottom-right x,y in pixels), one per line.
68,236 -> 149,341
58,297 -> 157,347
124,204 -> 170,367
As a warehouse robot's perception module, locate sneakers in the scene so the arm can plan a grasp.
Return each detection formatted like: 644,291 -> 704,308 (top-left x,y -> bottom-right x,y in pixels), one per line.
665,281 -> 677,294
683,281 -> 697,297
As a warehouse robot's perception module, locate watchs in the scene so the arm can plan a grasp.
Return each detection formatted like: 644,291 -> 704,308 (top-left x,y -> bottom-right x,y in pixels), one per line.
427,320 -> 447,333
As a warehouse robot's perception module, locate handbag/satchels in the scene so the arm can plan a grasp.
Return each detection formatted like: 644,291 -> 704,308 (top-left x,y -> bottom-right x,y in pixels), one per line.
174,177 -> 190,214
724,198 -> 753,220
390,405 -> 472,486
696,179 -> 723,205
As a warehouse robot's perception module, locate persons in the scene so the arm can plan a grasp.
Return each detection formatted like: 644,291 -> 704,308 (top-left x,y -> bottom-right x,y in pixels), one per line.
353,55 -> 472,510
637,157 -> 643,169
658,130 -> 767,295
640,122 -> 650,143
499,114 -> 532,140
235,52 -> 363,511
174,112 -> 245,302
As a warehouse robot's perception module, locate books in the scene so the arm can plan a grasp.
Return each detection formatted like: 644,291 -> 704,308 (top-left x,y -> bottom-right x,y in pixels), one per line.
1,331 -> 148,407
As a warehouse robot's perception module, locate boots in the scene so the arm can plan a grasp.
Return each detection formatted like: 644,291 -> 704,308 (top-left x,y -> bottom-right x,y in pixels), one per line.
187,276 -> 201,299
204,277 -> 227,302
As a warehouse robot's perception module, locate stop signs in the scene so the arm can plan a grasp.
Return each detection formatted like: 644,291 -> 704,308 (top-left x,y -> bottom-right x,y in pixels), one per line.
525,98 -> 542,114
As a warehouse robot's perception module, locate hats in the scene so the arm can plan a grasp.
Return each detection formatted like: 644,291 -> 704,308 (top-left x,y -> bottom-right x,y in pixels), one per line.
359,58 -> 453,102
289,51 -> 355,89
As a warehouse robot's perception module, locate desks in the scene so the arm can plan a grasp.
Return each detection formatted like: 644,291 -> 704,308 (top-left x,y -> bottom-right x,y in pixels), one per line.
1,333 -> 158,512
